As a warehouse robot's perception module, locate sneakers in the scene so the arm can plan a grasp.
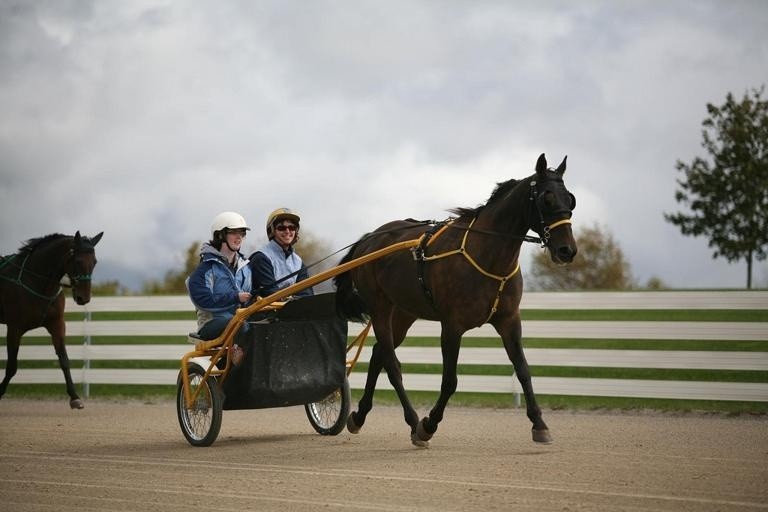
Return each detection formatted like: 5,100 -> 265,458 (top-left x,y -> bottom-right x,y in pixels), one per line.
231,344 -> 244,366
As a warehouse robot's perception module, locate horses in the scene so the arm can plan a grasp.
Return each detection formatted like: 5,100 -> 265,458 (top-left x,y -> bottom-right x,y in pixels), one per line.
0,227 -> 104,409
331,153 -> 578,451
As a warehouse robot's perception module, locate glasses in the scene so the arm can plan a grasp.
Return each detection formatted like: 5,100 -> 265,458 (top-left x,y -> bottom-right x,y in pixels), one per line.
274,225 -> 298,231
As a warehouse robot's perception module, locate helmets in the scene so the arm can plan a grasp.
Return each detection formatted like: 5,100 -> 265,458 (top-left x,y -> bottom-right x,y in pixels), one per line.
267,208 -> 300,228
213,212 -> 251,233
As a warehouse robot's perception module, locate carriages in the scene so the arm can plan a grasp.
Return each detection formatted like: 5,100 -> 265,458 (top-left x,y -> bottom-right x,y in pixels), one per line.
176,152 -> 577,450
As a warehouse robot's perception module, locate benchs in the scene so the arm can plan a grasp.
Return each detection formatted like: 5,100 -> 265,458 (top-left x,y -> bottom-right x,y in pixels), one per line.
186,332 -> 237,352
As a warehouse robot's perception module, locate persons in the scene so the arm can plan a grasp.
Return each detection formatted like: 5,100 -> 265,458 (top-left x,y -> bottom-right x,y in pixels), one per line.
185,211 -> 293,371
247,207 -> 315,321
